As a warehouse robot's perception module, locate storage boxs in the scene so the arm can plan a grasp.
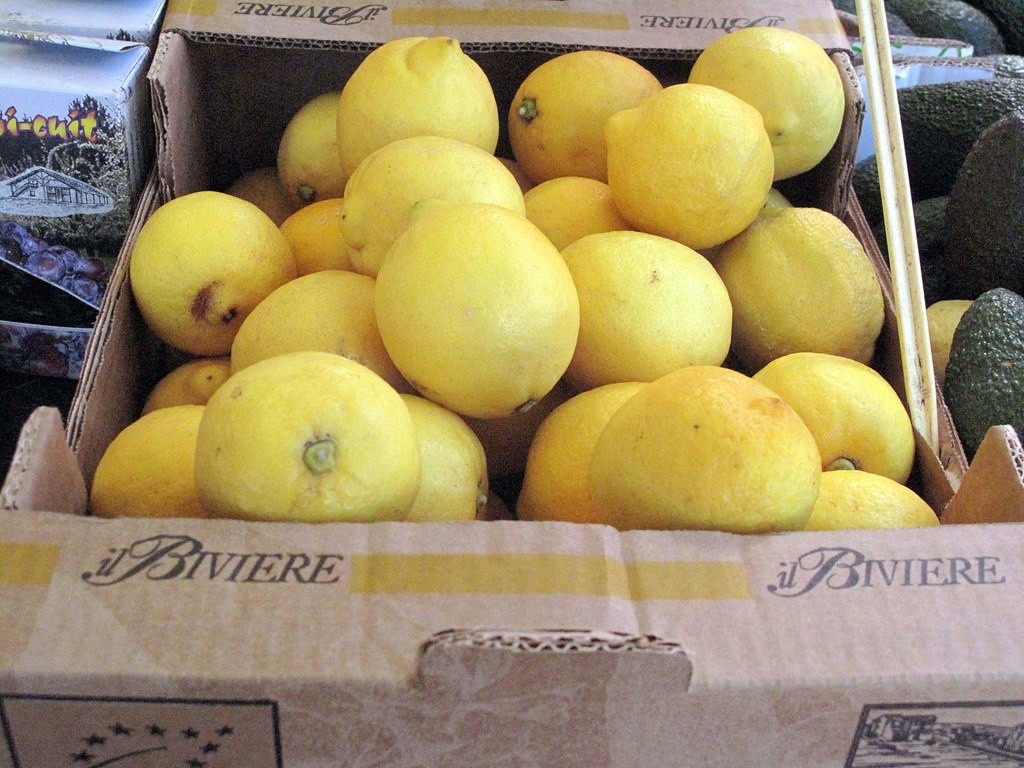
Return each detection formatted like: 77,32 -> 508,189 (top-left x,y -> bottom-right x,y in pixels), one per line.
0,0 -> 1024,768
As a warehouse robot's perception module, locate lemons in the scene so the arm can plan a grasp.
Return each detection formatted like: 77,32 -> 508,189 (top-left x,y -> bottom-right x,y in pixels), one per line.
86,26 -> 948,534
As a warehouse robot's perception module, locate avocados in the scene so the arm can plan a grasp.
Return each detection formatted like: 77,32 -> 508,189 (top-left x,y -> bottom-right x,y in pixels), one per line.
827,0 -> 1024,461
